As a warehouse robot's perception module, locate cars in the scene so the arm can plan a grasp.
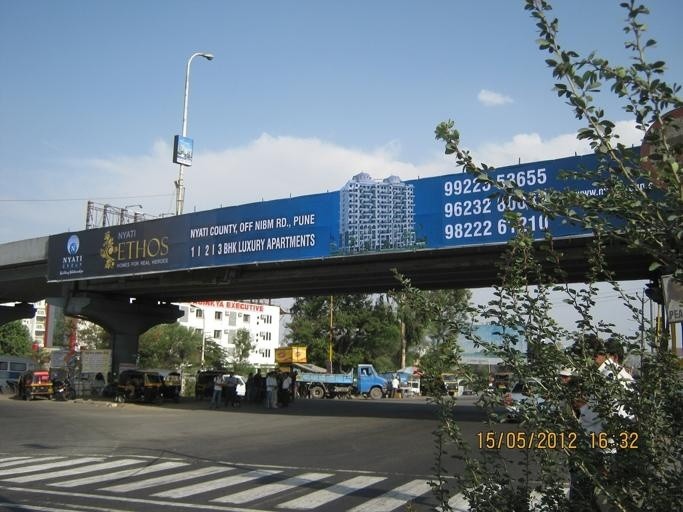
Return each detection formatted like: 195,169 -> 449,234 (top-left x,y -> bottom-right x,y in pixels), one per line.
506,380 -> 559,423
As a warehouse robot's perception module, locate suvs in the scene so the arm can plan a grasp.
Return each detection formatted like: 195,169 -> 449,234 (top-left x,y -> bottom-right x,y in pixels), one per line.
120,370 -> 163,401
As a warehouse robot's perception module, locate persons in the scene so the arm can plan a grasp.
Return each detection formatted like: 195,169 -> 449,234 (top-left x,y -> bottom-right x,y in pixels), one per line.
390,376 -> 399,398
559,333 -> 640,512
210,369 -> 298,410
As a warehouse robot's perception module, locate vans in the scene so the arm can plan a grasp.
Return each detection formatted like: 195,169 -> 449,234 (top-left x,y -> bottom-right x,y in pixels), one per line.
398,381 -> 420,396
195,373 -> 246,400
0,355 -> 42,394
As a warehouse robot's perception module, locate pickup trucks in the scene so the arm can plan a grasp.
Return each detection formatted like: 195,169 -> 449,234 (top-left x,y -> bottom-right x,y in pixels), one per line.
24,371 -> 54,400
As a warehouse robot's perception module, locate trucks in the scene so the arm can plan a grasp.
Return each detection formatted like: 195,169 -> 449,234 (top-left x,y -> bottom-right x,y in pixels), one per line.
438,372 -> 458,395
295,364 -> 389,400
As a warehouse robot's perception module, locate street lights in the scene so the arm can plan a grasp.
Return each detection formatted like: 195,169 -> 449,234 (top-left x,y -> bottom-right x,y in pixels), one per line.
173,52 -> 214,215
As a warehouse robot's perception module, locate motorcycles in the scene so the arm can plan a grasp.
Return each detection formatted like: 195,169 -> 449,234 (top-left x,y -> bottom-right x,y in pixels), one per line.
55,373 -> 76,400
